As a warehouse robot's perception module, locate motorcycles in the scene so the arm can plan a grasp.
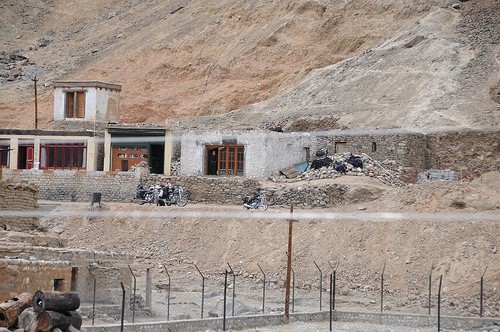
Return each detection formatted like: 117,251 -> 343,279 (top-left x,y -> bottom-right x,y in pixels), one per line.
241,186 -> 268,211
136,181 -> 162,205
157,184 -> 187,207
